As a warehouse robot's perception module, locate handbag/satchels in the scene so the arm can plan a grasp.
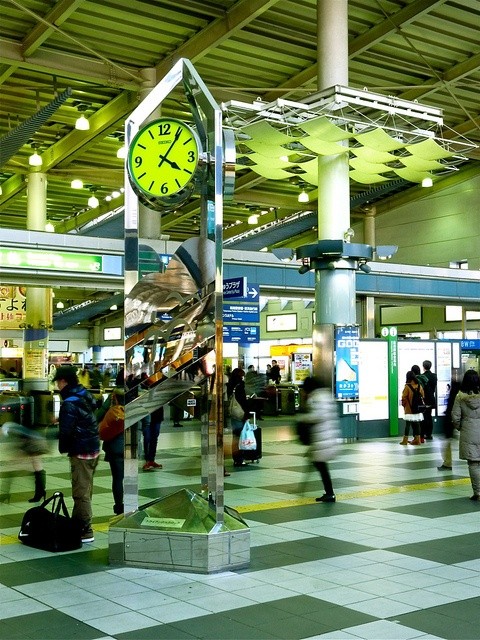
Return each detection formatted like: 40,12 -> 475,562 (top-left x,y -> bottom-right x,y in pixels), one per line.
98,392 -> 124,440
226,384 -> 245,421
238,420 -> 257,451
18,491 -> 82,552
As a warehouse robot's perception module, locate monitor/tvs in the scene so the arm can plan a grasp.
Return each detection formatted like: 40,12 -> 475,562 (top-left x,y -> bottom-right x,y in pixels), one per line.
379,304 -> 424,326
102,326 -> 122,342
444,305 -> 480,322
265,311 -> 298,332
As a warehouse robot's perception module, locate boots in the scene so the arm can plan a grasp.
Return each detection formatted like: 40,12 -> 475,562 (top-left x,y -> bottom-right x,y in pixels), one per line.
29,470 -> 46,502
0,471 -> 17,504
400,436 -> 408,445
410,435 -> 420,445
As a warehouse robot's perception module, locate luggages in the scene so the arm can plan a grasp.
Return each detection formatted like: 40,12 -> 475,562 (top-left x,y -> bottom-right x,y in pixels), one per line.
244,411 -> 262,463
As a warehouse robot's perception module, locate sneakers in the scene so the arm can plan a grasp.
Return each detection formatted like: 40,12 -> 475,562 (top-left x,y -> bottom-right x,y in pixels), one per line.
142,461 -> 153,471
81,529 -> 94,543
437,465 -> 452,471
153,462 -> 162,469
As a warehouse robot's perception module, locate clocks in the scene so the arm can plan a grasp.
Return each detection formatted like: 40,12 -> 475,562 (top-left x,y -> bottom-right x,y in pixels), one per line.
127,118 -> 202,213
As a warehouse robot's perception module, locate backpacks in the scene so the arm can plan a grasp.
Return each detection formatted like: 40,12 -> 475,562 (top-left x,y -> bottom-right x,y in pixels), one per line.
405,382 -> 427,414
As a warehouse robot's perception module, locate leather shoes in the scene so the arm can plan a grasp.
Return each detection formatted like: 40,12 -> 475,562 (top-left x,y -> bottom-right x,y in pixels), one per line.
316,494 -> 335,502
225,471 -> 231,476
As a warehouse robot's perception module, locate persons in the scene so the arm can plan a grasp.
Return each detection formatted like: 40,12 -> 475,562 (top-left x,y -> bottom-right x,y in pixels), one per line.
96,367 -> 124,423
437,442 -> 452,470
399,371 -> 425,445
78,364 -> 91,391
265,364 -> 272,384
227,367 -> 250,468
141,406 -> 162,470
290,375 -> 342,502
0,373 -> 46,505
270,359 -> 281,385
410,365 -> 429,442
168,394 -> 185,428
89,367 -> 101,390
51,366 -> 102,543
225,366 -> 232,400
421,360 -> 438,440
99,390 -> 123,515
451,369 -> 480,501
232,361 -> 245,387
246,364 -> 258,380
443,384 -> 451,414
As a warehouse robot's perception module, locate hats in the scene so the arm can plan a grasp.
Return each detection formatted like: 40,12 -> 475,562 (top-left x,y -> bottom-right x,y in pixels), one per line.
51,365 -> 76,382
